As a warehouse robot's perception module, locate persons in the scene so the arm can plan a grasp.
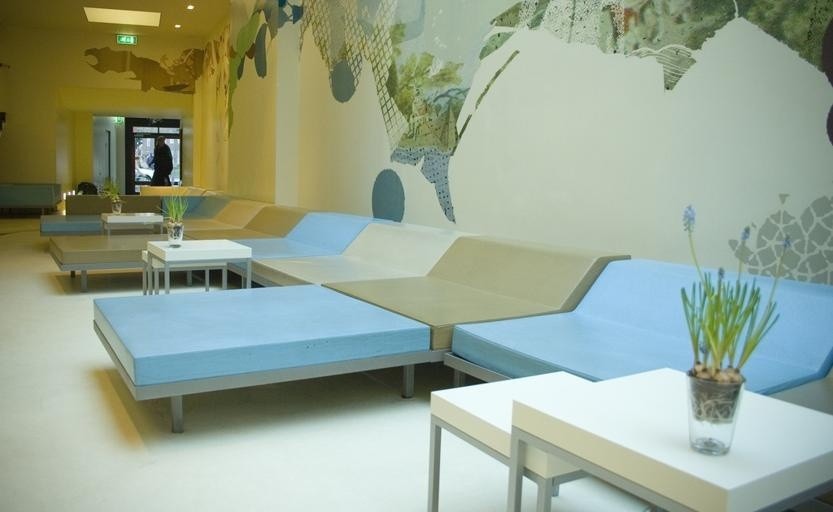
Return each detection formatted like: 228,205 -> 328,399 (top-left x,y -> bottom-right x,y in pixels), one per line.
149,136 -> 173,186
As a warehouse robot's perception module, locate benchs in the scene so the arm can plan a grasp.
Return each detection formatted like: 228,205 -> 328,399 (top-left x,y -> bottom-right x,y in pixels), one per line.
0,182 -> 61,217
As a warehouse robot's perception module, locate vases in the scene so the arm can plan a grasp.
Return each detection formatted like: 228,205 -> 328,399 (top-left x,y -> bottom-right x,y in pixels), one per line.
685,368 -> 744,455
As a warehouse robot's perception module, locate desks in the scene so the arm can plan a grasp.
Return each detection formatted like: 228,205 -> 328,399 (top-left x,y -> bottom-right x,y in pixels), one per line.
426,368 -> 832,511
140,249 -> 228,295
147,239 -> 253,294
99,211 -> 164,235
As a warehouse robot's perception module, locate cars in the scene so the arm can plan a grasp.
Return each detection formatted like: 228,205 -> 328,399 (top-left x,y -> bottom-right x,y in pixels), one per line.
134,155 -> 154,182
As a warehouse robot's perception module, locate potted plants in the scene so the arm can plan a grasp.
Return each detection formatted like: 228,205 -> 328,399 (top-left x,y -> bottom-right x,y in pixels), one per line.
155,181 -> 189,248
102,176 -> 123,215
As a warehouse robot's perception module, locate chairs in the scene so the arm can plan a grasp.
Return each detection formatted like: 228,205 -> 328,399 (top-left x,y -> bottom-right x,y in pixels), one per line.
94,234 -> 630,432
441,259 -> 833,400
155,193 -> 271,232
226,212 -> 464,286
49,204 -> 311,292
39,187 -> 205,236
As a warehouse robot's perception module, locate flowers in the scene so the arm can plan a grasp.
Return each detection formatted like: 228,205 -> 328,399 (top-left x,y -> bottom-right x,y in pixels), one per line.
677,204 -> 792,383
